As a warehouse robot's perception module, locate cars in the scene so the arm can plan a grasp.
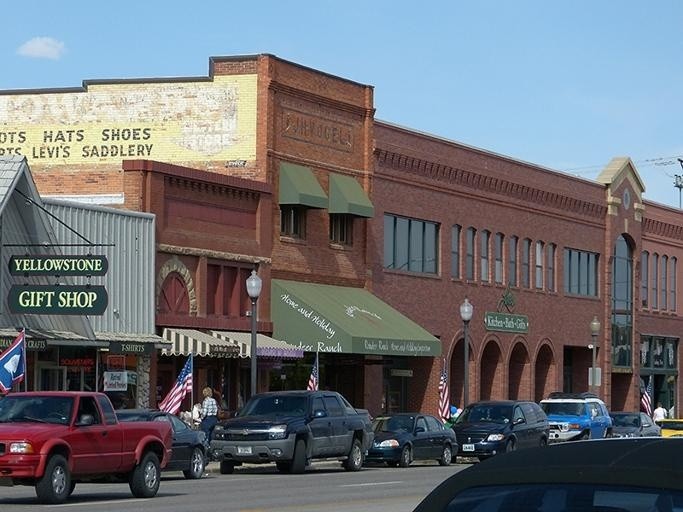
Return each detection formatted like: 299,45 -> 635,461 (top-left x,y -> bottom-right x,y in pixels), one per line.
607,411 -> 661,441
360,412 -> 459,470
114,407 -> 213,480
412,436 -> 682,512
653,417 -> 682,438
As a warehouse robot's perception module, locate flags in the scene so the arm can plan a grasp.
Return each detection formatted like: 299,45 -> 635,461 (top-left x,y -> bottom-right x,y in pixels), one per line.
437,367 -> 451,423
306,359 -> 318,391
158,355 -> 193,415
640,378 -> 652,417
0,329 -> 26,396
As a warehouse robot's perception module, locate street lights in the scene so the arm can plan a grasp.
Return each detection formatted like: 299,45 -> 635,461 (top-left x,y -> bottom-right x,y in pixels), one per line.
245,268 -> 273,399
587,314 -> 603,396
457,294 -> 480,409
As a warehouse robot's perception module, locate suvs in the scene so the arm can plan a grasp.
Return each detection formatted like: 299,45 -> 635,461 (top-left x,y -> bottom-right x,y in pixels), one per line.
535,391 -> 614,445
448,398 -> 552,464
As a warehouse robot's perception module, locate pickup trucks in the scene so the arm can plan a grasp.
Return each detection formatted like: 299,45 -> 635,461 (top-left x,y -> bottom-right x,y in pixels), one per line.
0,391 -> 173,505
208,389 -> 375,476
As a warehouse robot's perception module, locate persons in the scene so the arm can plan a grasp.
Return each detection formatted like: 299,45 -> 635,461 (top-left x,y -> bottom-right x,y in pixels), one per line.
450,406 -> 464,417
198,387 -> 218,466
652,402 -> 668,427
